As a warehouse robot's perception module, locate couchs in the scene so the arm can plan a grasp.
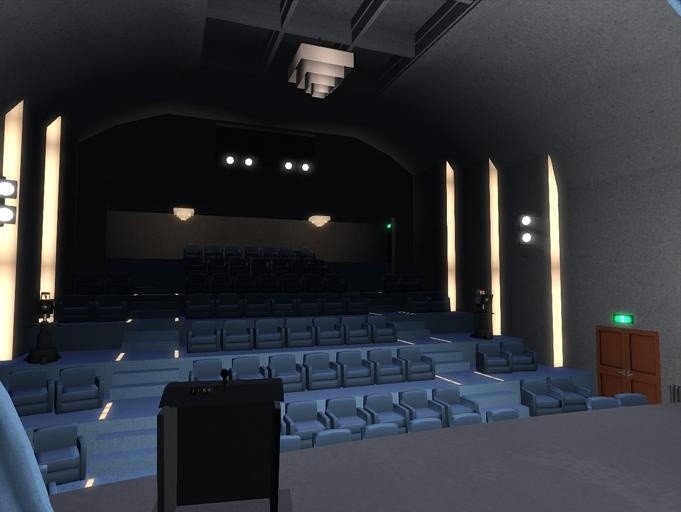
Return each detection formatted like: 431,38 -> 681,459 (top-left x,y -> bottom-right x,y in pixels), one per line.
57,292 -> 185,322
183,244 -> 328,292
26,422 -> 87,486
585,393 -> 647,413
280,386 -> 482,453
520,376 -> 591,417
486,408 -> 520,424
185,293 -> 450,319
7,364 -> 105,417
186,319 -> 398,353
189,347 -> 436,392
476,341 -> 538,374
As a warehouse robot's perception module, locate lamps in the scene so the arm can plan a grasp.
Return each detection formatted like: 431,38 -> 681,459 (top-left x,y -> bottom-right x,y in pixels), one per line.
287,43 -> 354,100
173,206 -> 195,222
309,216 -> 332,228
